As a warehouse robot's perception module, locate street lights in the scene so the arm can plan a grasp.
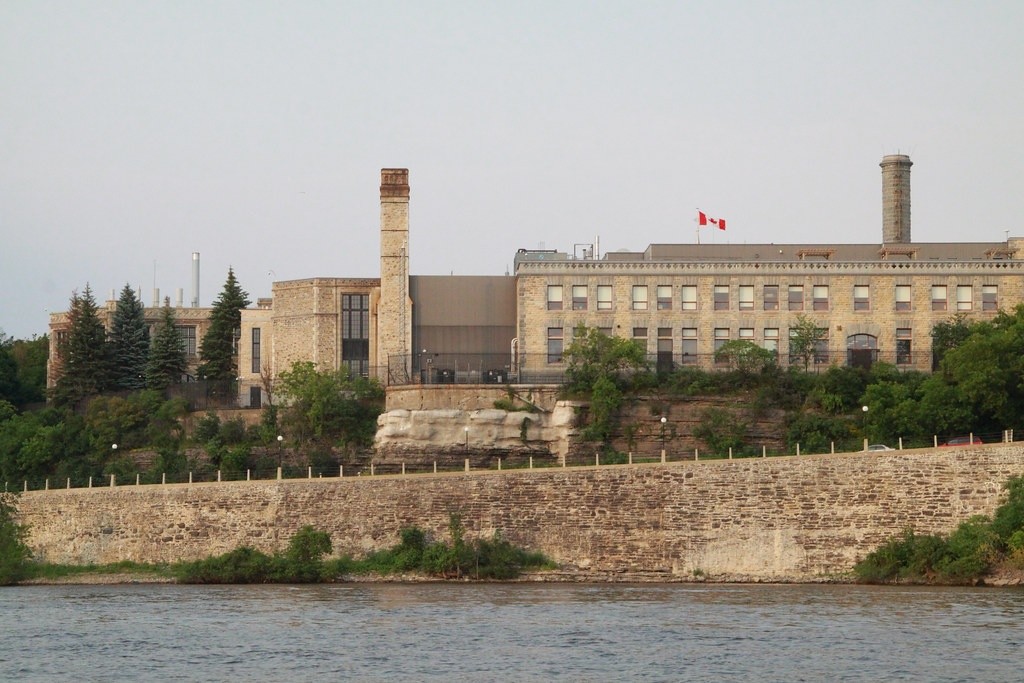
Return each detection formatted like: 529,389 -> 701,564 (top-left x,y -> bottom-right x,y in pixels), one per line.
277,435 -> 284,467
661,416 -> 667,450
464,426 -> 470,460
862,405 -> 869,439
112,444 -> 118,475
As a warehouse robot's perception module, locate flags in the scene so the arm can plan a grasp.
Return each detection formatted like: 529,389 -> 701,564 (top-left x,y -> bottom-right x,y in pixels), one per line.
699,211 -> 726,231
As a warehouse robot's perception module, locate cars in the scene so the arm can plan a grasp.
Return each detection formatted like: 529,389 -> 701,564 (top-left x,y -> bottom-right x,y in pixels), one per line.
859,444 -> 896,452
938,436 -> 983,448
